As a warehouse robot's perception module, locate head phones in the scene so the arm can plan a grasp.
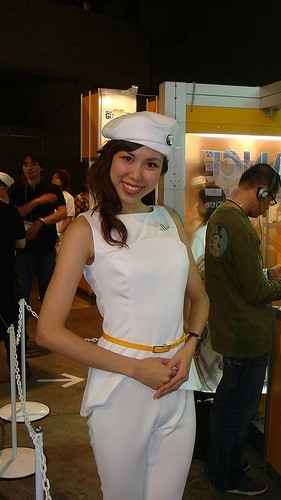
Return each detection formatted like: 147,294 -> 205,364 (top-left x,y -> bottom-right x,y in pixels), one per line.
257,172 -> 278,200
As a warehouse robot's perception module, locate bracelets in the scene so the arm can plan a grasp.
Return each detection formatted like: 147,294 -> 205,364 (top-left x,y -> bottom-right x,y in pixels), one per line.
187,332 -> 201,339
39,217 -> 47,226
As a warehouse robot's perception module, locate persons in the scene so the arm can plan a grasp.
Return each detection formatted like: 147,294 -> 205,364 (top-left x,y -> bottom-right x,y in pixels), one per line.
204,164 -> 281,495
0,111 -> 227,500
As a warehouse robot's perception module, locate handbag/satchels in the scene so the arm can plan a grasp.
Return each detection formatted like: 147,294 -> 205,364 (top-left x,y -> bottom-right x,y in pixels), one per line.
23,222 -> 33,232
199,327 -> 225,395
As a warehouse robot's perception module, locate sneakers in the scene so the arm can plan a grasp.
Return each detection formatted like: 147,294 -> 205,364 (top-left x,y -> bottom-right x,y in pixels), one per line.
240,459 -> 252,469
208,476 -> 268,497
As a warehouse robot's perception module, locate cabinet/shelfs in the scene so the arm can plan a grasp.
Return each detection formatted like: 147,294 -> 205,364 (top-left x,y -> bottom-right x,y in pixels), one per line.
262,306 -> 281,483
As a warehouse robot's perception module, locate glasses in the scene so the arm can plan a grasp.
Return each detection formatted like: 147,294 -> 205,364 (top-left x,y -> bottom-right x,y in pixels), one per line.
257,186 -> 277,206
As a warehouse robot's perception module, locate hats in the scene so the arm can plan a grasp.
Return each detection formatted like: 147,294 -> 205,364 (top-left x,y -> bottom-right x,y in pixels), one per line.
99,111 -> 180,159
0,172 -> 14,187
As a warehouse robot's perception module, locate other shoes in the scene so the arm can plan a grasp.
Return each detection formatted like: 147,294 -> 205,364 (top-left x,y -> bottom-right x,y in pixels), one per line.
27,365 -> 32,377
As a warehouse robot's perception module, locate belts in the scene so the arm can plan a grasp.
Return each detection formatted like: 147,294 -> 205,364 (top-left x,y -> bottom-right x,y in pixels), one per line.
102,330 -> 191,353
